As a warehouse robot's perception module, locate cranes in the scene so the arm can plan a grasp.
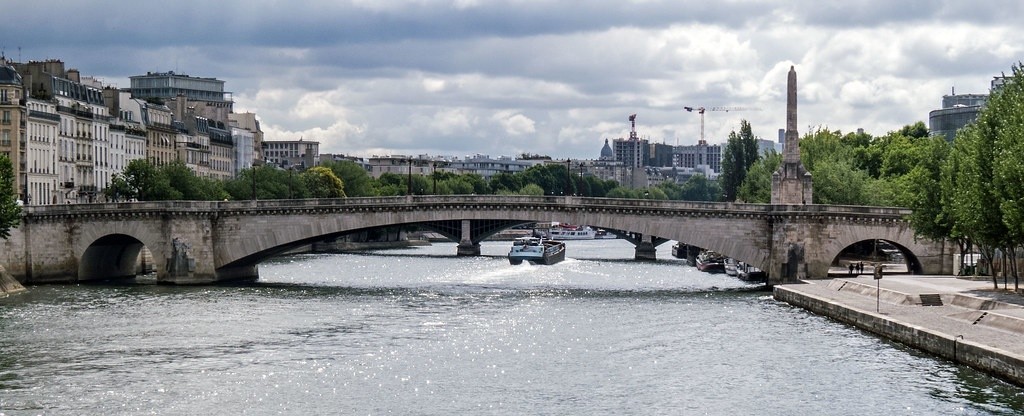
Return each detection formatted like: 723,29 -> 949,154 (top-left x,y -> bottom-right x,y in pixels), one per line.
682,104 -> 764,140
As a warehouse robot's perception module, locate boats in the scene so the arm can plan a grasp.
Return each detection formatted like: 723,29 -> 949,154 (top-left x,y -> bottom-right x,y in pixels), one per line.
507,236 -> 565,266
695,249 -> 727,272
595,228 -> 605,239
538,227 -> 597,240
736,261 -> 764,282
723,258 -> 739,276
491,230 -> 531,240
559,223 -> 579,230
672,241 -> 687,258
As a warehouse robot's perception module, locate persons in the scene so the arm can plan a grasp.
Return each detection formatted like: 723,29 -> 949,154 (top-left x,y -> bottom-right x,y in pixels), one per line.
520,239 -> 526,245
849,262 -> 864,274
573,192 -> 585,197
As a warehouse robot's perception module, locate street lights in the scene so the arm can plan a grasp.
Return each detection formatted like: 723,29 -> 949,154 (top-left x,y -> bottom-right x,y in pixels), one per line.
289,167 -> 294,199
579,163 -> 583,196
433,162 -> 437,195
408,156 -> 413,194
251,162 -> 257,208
566,157 -> 572,195
